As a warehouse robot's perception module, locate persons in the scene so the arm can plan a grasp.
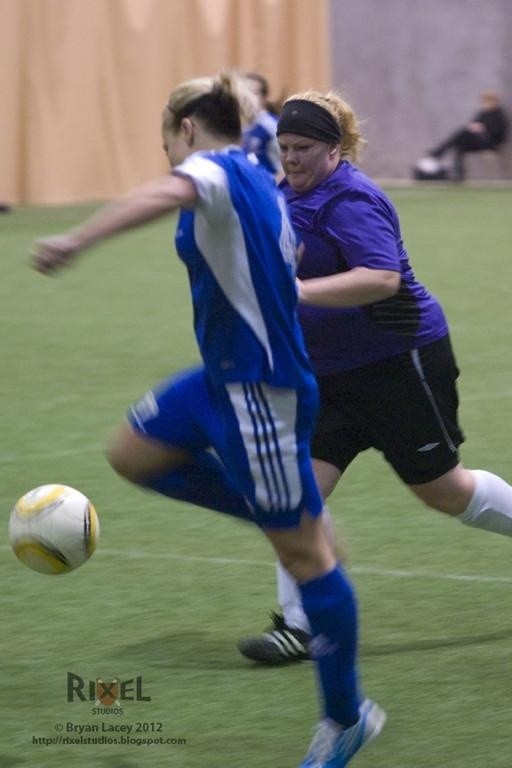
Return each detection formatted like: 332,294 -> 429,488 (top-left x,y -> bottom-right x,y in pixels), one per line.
232,85 -> 510,672
232,70 -> 295,188
26,63 -> 389,768
411,92 -> 508,180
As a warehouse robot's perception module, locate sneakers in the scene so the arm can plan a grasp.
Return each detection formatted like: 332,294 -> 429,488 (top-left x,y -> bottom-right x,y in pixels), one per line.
236,608 -> 318,665
299,696 -> 388,767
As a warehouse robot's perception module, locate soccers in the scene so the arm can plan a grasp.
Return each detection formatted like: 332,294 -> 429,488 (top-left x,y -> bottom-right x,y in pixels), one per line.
9,484 -> 99,575
